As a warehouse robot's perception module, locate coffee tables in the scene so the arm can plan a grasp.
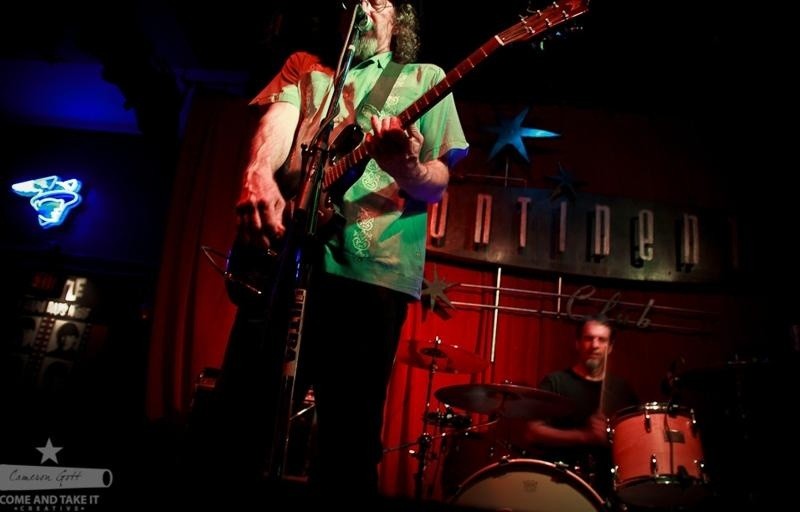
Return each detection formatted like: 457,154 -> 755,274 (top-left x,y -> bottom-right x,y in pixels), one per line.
676,359 -> 789,393
434,383 -> 575,422
393,338 -> 486,375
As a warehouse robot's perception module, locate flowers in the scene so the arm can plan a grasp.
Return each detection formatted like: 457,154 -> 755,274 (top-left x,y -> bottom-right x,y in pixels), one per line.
353,0 -> 374,32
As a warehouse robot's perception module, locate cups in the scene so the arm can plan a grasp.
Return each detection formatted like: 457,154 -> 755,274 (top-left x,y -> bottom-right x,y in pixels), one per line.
342,0 -> 392,13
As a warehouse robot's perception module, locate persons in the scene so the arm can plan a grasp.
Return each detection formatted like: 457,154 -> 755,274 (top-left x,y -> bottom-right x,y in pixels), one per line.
526,316 -> 639,495
44,322 -> 80,360
191,0 -> 471,509
15,316 -> 36,353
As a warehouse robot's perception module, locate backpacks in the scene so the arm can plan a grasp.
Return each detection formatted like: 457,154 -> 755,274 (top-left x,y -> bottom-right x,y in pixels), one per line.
609,401 -> 713,507
438,456 -> 611,511
